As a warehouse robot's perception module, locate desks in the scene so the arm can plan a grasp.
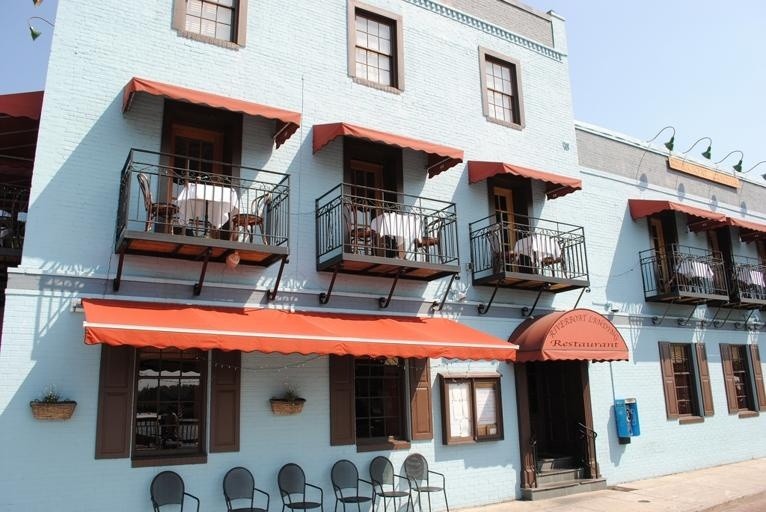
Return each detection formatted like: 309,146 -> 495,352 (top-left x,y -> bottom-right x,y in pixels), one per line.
663,254 -> 766,298
174,182 -> 241,239
512,233 -> 563,276
370,212 -> 423,261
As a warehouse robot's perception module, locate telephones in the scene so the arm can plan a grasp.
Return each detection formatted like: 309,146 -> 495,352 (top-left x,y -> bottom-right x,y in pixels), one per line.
625,405 -> 631,432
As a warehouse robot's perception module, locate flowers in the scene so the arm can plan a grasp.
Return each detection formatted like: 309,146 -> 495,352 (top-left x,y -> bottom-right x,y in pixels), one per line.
279,380 -> 303,400
37,381 -> 64,402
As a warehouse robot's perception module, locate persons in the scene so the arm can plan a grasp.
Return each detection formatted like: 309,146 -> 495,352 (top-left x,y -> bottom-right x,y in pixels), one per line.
160,404 -> 180,446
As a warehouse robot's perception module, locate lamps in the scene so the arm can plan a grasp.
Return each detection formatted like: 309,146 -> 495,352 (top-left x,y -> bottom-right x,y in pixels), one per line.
448,288 -> 467,302
593,298 -> 620,315
645,125 -> 766,182
24,15 -> 55,42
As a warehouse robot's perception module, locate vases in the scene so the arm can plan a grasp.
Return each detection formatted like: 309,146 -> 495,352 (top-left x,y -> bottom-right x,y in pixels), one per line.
28,400 -> 78,421
269,398 -> 307,416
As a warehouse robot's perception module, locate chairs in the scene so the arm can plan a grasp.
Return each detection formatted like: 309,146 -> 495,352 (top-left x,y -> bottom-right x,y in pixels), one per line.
150,471 -> 199,512
231,192 -> 271,246
413,218 -> 446,264
540,239 -> 568,279
484,230 -> 518,272
278,463 -> 324,512
330,453 -> 449,512
223,467 -> 270,512
340,198 -> 378,256
135,169 -> 180,234
